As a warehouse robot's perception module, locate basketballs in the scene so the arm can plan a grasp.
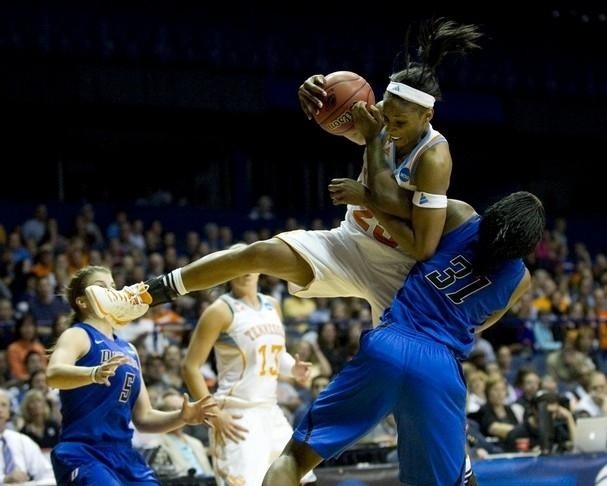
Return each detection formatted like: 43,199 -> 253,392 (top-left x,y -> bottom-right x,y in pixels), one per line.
313,70 -> 374,137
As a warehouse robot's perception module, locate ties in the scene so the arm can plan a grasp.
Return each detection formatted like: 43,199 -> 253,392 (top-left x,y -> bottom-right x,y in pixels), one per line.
0,437 -> 14,475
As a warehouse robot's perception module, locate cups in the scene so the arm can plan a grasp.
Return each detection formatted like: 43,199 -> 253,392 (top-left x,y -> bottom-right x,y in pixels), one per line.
516,438 -> 530,452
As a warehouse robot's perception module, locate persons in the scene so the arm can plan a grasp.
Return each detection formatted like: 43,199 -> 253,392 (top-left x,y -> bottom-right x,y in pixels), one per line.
83,15 -> 482,486
258,179 -> 547,486
462,220 -> 607,456
0,190 -> 398,485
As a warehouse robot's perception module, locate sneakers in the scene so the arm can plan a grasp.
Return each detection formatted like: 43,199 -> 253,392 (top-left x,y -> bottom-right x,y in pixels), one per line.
85,281 -> 153,330
